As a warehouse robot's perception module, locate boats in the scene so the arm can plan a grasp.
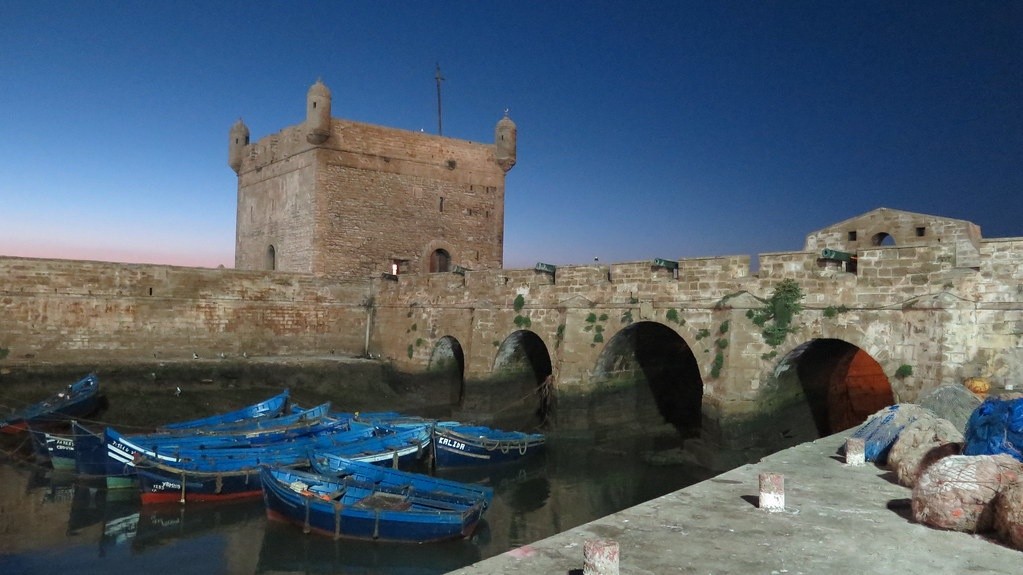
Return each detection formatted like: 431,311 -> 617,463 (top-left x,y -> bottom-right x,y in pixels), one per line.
1,385 -> 548,511
0,368 -> 107,445
307,446 -> 496,515
258,461 -> 489,548
